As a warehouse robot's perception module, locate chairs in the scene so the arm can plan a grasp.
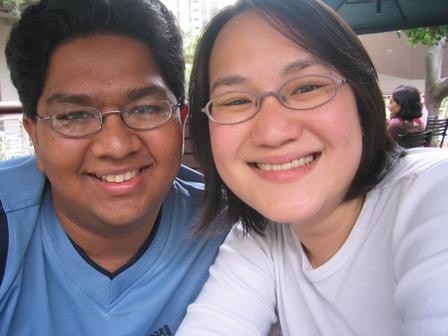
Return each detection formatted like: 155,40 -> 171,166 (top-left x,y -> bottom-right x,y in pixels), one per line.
425,117 -> 448,148
181,111 -> 207,174
396,129 -> 434,150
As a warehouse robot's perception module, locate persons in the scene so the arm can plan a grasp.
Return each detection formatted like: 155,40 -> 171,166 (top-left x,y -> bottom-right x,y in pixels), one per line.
0,0 -> 448,336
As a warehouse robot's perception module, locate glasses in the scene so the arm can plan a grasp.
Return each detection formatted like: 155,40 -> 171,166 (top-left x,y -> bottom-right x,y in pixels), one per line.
197,74 -> 349,126
33,100 -> 184,138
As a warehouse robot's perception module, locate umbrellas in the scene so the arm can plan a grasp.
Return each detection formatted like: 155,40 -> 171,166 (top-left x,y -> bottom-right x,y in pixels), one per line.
322,0 -> 448,35
